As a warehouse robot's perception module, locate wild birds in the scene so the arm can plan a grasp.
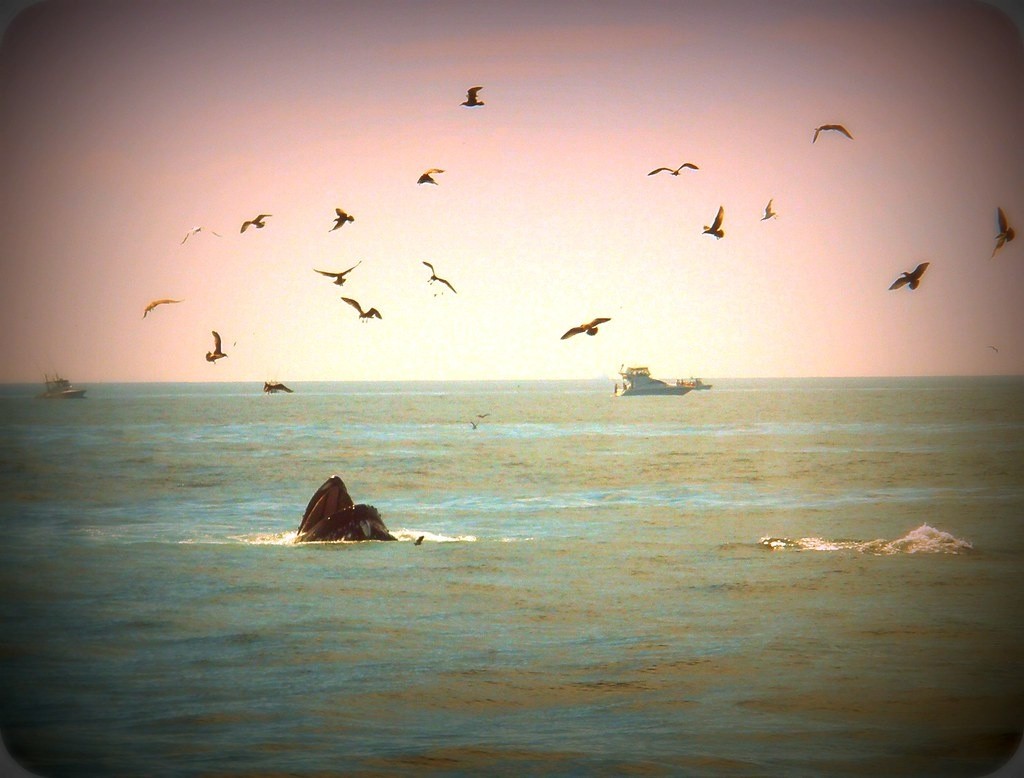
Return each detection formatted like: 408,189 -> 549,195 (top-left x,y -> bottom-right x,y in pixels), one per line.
422,262 -> 456,295
413,536 -> 424,546
988,206 -> 1015,260
701,206 -> 726,239
327,208 -> 355,232
180,226 -> 220,244
341,297 -> 382,320
262,381 -> 293,394
459,86 -> 484,107
760,198 -> 776,220
560,317 -> 611,339
206,331 -> 228,364
812,124 -> 855,143
240,214 -> 272,234
313,261 -> 362,287
142,299 -> 181,319
648,163 -> 700,177
470,414 -> 491,430
887,262 -> 930,291
416,168 -> 444,186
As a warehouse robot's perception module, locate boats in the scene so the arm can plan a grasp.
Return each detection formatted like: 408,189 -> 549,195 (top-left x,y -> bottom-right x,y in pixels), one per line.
613,362 -> 695,396
676,376 -> 714,391
40,372 -> 91,399
